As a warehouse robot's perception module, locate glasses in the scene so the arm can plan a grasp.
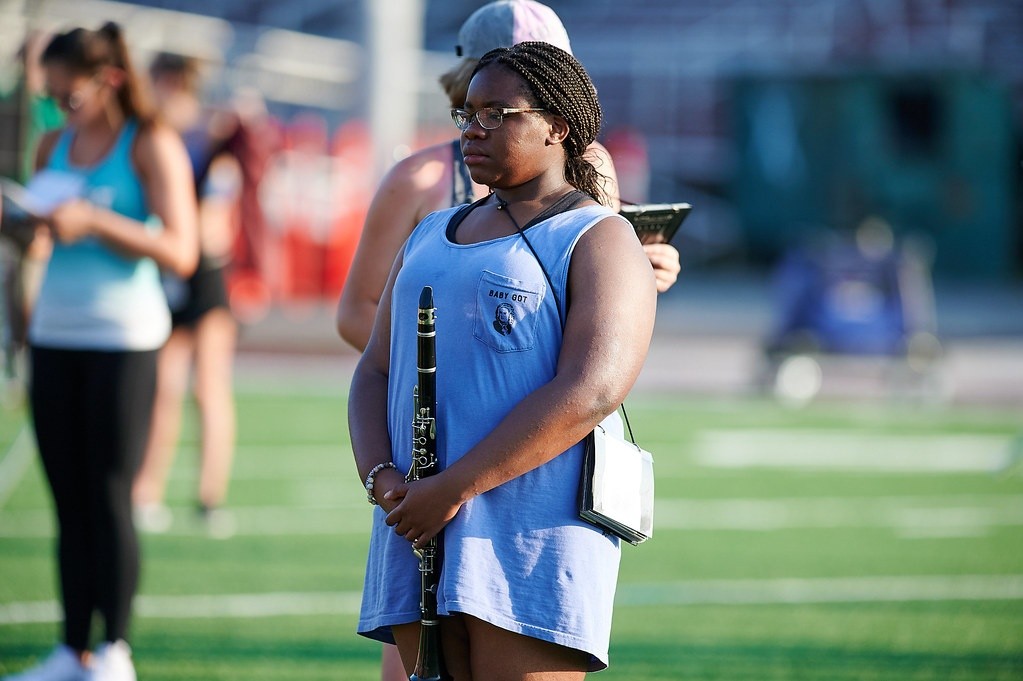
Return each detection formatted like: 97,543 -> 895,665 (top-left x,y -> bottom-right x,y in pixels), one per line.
38,76 -> 110,114
450,107 -> 548,130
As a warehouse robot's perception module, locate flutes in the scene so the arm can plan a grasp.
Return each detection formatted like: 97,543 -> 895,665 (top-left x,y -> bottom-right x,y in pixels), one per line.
402,285 -> 455,681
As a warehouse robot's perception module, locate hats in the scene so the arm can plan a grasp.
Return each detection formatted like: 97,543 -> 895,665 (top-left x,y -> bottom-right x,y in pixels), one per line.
454,0 -> 574,59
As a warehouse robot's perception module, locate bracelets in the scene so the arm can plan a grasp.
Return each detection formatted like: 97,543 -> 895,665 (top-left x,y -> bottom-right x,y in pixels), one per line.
366,461 -> 395,504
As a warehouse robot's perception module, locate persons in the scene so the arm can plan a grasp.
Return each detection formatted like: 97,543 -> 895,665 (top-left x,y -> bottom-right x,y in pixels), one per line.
1,21 -> 200,681
336,0 -> 680,680
131,52 -> 253,538
350,42 -> 657,680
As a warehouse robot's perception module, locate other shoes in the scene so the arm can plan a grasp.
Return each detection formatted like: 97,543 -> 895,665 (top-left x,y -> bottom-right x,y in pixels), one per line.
83,638 -> 136,681
4,644 -> 94,681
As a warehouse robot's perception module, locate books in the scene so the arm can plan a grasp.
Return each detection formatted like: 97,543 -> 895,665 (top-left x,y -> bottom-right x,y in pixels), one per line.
619,201 -> 693,246
